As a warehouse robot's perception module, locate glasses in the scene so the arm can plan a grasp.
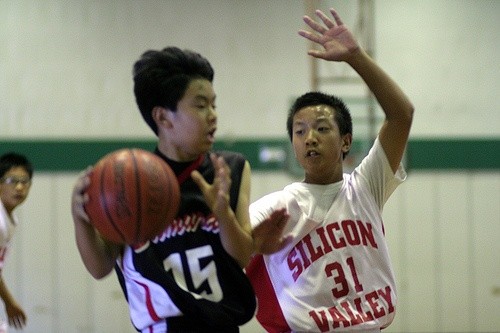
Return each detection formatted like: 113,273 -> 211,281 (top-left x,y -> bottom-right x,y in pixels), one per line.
1,175 -> 31,188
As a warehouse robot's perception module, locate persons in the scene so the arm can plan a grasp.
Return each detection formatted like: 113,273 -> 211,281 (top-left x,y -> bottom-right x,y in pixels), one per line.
72,45 -> 261,333
0,152 -> 36,330
246,8 -> 416,332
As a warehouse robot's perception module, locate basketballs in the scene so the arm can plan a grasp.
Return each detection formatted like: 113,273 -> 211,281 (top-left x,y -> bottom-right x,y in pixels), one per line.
82,146 -> 181,246
